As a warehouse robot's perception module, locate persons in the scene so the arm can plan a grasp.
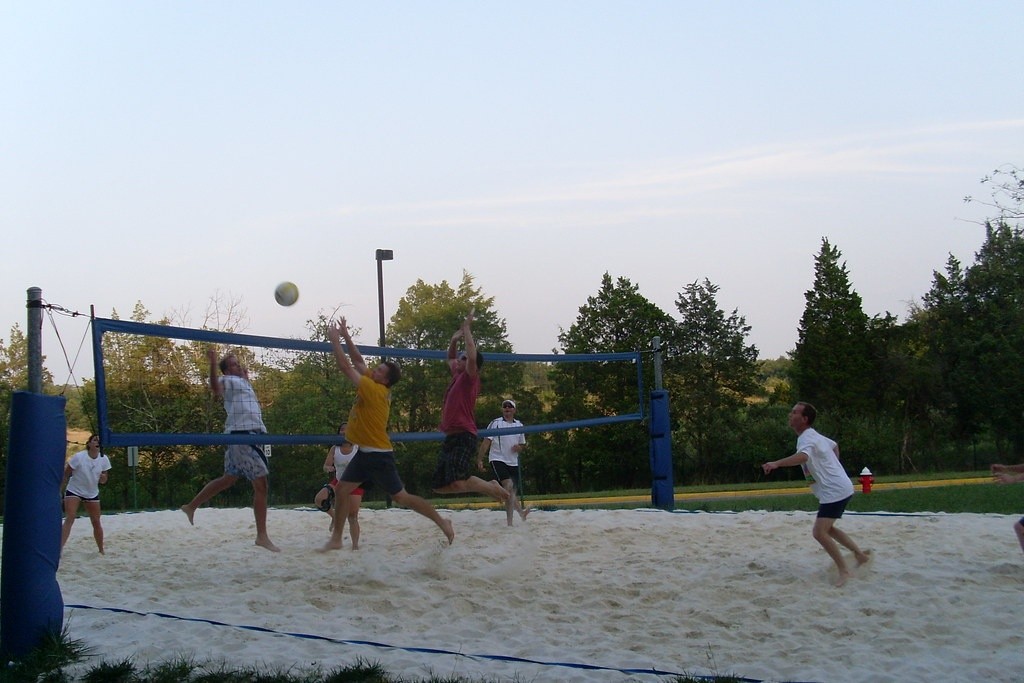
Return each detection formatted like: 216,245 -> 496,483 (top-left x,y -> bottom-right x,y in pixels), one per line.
992,464 -> 1024,551
61,434 -> 112,555
181,347 -> 281,554
430,309 -> 511,502
317,316 -> 454,552
314,422 -> 364,550
477,399 -> 530,526
762,402 -> 870,577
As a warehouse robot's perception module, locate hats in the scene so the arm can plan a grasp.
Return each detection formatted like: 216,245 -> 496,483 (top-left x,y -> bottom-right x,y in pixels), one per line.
502,400 -> 516,409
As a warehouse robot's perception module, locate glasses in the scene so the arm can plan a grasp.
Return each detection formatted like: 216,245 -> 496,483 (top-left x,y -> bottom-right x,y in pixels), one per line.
502,404 -> 513,408
460,355 -> 467,361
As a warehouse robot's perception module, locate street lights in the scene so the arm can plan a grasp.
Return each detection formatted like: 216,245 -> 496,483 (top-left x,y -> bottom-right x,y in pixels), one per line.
376,248 -> 394,362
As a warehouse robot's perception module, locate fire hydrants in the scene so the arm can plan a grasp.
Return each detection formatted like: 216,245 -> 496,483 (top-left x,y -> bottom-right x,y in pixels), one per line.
856,463 -> 876,497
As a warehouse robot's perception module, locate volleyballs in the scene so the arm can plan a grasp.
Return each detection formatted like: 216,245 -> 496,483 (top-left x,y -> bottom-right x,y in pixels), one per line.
274,281 -> 300,308
